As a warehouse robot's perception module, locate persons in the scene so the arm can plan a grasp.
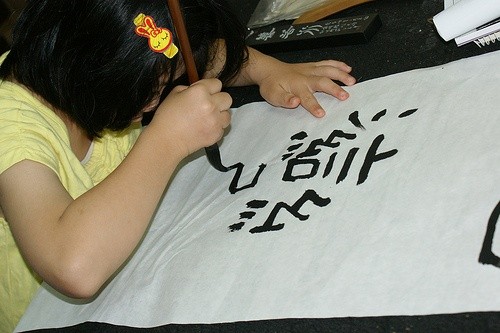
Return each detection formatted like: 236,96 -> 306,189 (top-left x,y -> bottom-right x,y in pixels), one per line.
0,0 -> 355,333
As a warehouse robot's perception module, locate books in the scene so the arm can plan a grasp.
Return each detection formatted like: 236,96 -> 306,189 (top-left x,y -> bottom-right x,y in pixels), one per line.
433,0 -> 500,46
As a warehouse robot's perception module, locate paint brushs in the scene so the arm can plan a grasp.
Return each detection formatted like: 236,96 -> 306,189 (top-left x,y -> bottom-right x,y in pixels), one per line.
169,0 -> 229,172
